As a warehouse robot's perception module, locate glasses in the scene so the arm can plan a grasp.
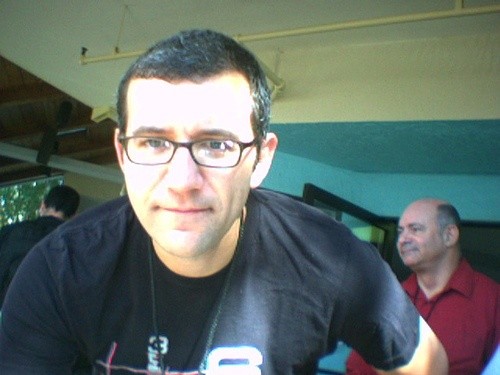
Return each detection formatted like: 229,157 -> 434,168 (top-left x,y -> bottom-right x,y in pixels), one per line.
116,133 -> 261,167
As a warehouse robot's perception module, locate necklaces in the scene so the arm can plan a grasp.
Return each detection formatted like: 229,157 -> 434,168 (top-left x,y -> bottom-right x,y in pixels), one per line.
147,208 -> 245,375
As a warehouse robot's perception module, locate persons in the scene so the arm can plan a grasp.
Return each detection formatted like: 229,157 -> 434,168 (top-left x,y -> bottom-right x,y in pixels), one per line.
346,199 -> 500,375
0,29 -> 449,375
0,185 -> 80,313
